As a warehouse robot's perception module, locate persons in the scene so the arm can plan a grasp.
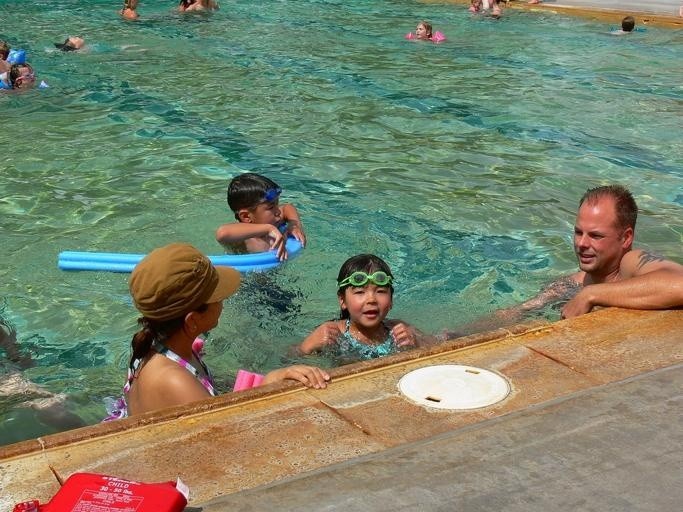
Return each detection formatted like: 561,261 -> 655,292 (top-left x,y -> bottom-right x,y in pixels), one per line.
8,62 -> 35,90
441,181 -> 682,342
611,14 -> 636,36
278,252 -> 438,367
0,40 -> 11,75
178,1 -> 219,13
0,318 -> 88,434
61,35 -> 85,51
212,172 -> 307,262
98,241 -> 330,423
468,0 -> 540,19
414,21 -> 433,42
117,0 -> 140,19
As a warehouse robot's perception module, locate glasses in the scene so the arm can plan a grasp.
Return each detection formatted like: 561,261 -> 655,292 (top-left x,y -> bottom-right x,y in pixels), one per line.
258,186 -> 282,204
338,272 -> 393,287
16,72 -> 34,81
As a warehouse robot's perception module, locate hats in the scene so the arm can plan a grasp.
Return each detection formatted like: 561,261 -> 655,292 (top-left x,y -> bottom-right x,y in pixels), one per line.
128,243 -> 241,321
